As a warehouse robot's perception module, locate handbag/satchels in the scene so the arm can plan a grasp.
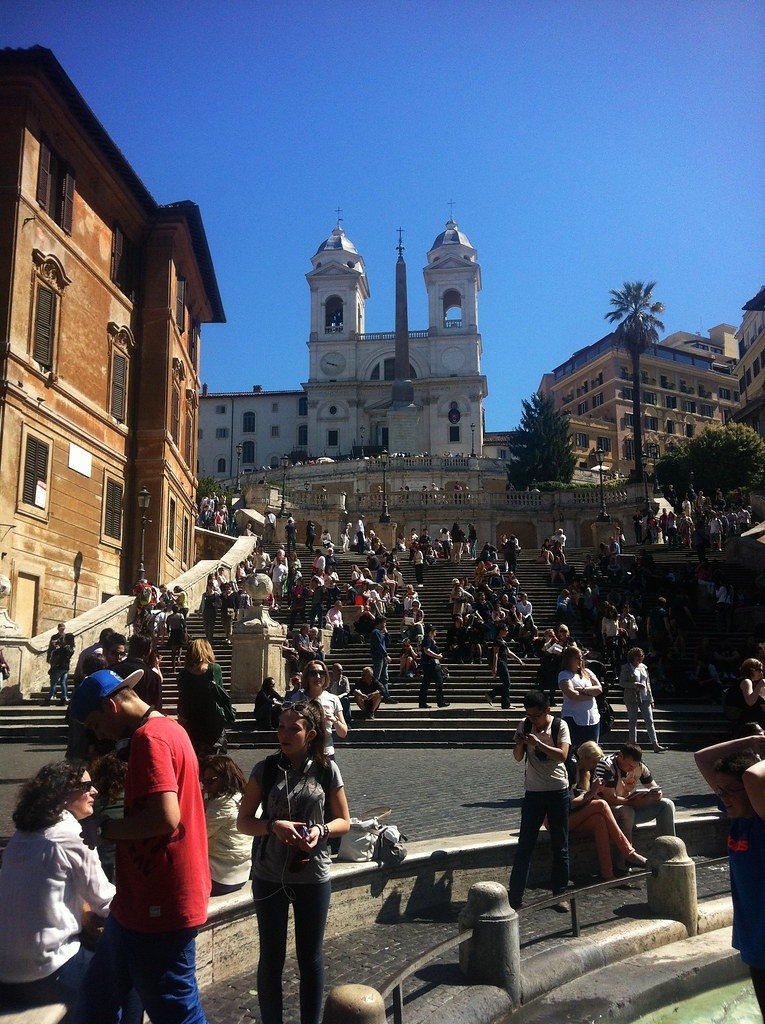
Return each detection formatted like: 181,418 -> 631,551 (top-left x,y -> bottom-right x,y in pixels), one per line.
596,685 -> 615,737
441,667 -> 449,682
200,663 -> 236,728
338,817 -> 407,870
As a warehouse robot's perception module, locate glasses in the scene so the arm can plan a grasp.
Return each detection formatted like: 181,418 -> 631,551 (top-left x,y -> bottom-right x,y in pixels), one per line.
525,709 -> 546,718
206,775 -> 219,783
112,650 -> 126,657
714,786 -> 745,797
310,670 -> 325,677
281,701 -> 306,712
79,781 -> 94,794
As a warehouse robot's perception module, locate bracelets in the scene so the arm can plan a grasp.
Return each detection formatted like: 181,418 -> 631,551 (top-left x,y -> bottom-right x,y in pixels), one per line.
581,687 -> 585,693
310,823 -> 330,844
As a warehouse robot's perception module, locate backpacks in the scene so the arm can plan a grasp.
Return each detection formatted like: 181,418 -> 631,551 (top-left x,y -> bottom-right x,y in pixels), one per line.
523,715 -> 577,791
139,587 -> 152,605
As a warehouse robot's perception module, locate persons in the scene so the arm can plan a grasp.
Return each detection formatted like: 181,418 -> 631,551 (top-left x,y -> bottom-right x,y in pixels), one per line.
236,700 -> 351,1024
0,627 -> 255,1024
0,451 -> 765,753
297,660 -> 347,854
509,646 -> 676,913
693,659 -> 765,1023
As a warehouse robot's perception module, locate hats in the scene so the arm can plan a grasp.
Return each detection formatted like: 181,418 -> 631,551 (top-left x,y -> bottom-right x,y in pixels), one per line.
424,623 -> 438,632
452,578 -> 460,584
292,676 -> 302,683
71,669 -> 144,722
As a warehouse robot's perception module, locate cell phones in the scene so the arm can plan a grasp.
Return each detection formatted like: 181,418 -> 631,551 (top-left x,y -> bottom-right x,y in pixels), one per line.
647,787 -> 663,793
516,733 -> 525,739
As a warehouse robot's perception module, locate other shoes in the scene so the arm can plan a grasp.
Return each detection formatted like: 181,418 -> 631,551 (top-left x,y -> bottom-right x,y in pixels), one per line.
552,900 -> 570,912
40,698 -> 50,706
419,705 -> 431,708
385,699 -> 399,704
508,705 -> 515,709
654,746 -> 668,752
56,700 -> 66,706
438,703 -> 450,708
485,692 -> 493,706
625,859 -> 646,868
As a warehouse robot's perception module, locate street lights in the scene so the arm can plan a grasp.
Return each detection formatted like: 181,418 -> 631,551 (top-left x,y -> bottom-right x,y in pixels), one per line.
594,448 -> 610,522
278,455 -> 288,518
470,423 -> 477,457
131,486 -> 151,595
234,442 -> 243,494
379,447 -> 391,524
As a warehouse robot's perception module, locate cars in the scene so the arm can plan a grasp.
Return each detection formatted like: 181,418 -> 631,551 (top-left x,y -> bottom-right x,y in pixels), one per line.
315,458 -> 335,464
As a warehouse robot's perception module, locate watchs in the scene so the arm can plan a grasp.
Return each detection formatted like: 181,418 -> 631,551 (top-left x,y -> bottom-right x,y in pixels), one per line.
334,715 -> 340,724
266,818 -> 277,840
96,819 -> 111,841
